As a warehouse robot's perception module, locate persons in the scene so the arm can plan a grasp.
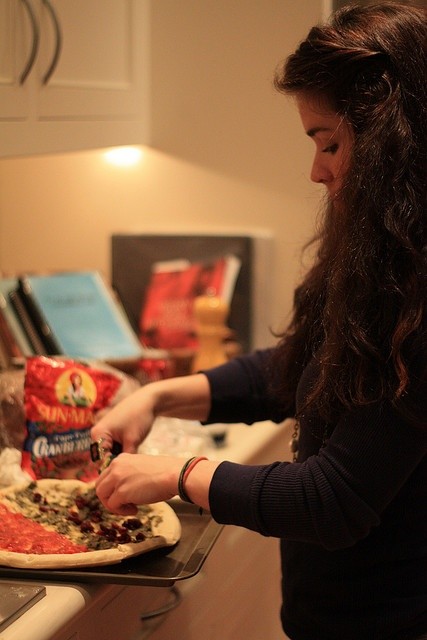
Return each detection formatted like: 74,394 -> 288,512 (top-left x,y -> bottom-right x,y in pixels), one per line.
88,2 -> 426,640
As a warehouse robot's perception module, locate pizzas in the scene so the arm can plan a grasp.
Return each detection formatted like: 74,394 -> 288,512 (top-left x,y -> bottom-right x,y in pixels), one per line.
0,476 -> 183,570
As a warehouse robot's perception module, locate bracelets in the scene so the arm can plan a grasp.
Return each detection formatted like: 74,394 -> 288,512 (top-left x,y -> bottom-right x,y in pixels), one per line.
178,456 -> 208,503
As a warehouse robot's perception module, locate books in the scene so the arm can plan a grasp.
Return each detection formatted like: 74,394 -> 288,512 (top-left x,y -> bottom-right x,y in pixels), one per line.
0,268 -> 142,363
139,254 -> 242,379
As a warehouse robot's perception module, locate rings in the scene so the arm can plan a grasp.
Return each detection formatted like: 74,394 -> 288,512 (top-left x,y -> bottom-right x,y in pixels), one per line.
98,438 -> 110,460
